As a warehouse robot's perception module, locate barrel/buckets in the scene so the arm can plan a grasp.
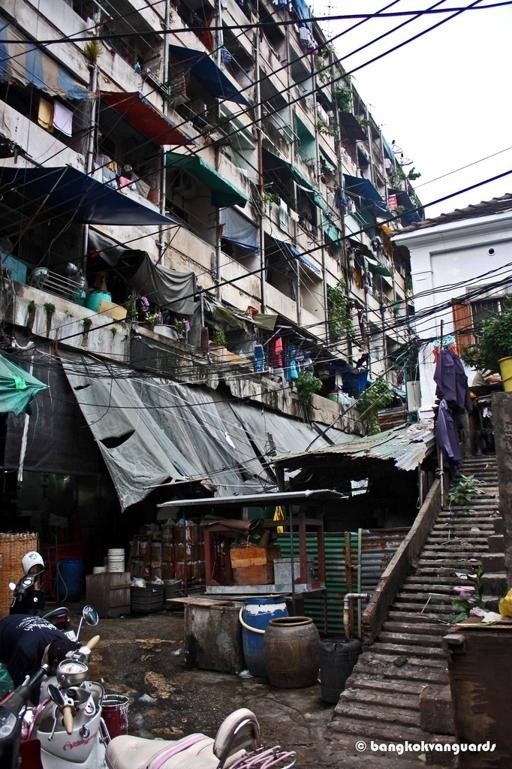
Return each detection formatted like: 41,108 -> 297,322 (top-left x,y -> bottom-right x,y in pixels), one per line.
107,547 -> 126,574
238,597 -> 293,679
100,694 -> 129,740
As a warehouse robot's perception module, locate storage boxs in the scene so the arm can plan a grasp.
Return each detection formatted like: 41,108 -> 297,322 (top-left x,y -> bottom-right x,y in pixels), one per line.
230,544 -> 280,584
273,558 -> 314,585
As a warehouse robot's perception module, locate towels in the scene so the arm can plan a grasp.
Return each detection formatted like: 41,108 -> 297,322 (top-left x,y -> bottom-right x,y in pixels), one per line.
51,99 -> 74,138
37,97 -> 54,133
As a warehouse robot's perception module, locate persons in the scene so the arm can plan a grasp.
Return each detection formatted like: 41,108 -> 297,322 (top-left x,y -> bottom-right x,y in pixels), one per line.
0,612 -> 82,703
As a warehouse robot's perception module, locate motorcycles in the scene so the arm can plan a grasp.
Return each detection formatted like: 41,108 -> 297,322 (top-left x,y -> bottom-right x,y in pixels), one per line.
9,551 -> 71,644
26,634 -> 298,769
0,664 -> 49,769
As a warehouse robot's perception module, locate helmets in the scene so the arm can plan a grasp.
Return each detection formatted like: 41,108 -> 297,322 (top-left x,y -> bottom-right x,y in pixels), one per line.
22,552 -> 44,575
